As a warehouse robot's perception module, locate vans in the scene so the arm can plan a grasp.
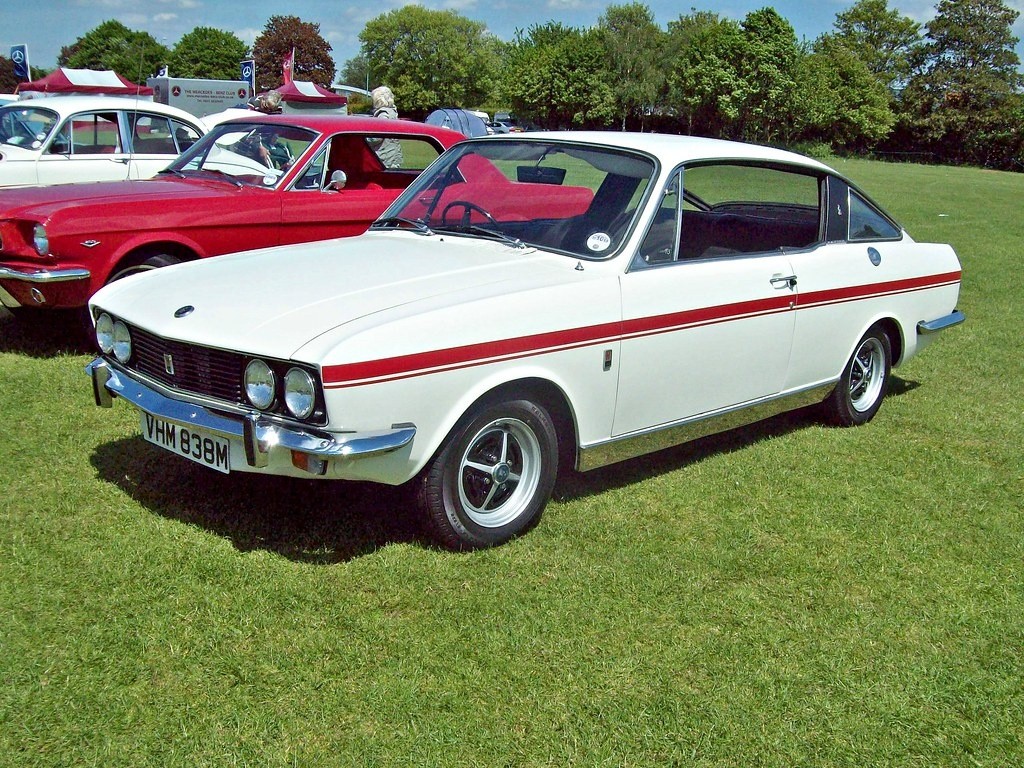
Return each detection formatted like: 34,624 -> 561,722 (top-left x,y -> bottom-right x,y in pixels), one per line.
470,111 -> 490,123
494,112 -> 543,131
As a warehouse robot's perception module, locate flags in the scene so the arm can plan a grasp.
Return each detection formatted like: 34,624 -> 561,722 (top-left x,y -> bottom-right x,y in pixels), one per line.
283,52 -> 291,85
157,68 -> 166,79
10,45 -> 28,78
240,60 -> 253,87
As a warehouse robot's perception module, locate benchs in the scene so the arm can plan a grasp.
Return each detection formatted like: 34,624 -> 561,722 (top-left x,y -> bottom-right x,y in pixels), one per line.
133,137 -> 202,155
605,205 -> 819,259
333,169 -> 452,189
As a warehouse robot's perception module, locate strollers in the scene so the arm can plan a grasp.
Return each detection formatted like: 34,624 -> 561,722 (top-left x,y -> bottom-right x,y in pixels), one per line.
251,138 -> 323,190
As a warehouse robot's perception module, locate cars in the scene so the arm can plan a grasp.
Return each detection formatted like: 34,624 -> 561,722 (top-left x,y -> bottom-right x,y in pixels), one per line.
0,95 -> 297,193
0,114 -> 594,354
486,122 -> 525,135
0,93 -> 93,154
83,131 -> 967,553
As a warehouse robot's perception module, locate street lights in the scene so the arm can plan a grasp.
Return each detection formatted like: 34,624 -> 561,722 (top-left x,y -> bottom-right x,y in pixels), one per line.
363,52 -> 368,98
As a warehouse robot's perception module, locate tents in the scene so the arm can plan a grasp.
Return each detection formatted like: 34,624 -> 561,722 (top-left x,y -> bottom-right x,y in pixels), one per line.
424,108 -> 489,140
255,80 -> 348,117
17,67 -> 152,148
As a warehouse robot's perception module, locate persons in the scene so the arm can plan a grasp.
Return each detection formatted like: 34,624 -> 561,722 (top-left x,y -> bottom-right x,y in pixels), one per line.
233,89 -> 284,169
365,86 -> 405,170
